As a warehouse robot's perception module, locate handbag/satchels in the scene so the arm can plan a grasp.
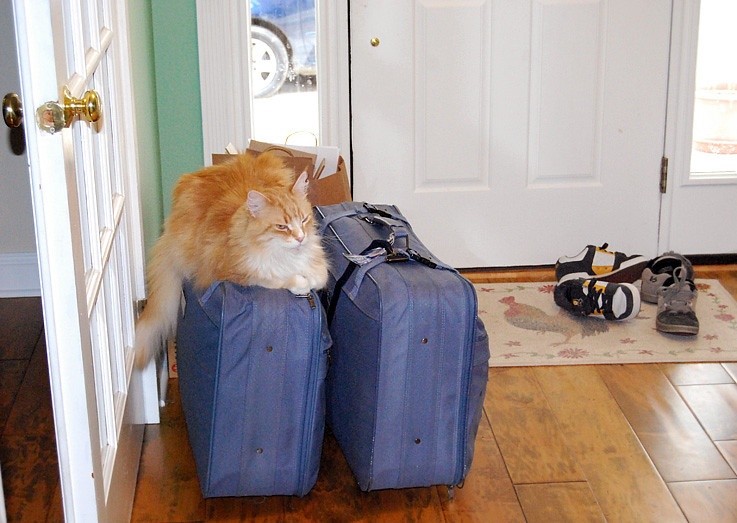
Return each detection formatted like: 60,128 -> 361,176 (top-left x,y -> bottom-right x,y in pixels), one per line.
177,268 -> 333,499
312,201 -> 492,493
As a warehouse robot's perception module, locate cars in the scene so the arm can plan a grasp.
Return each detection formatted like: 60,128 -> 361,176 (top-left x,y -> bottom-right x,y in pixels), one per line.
251,0 -> 317,99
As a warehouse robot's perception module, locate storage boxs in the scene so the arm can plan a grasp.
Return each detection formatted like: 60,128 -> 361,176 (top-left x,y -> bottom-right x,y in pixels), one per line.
211,139 -> 351,208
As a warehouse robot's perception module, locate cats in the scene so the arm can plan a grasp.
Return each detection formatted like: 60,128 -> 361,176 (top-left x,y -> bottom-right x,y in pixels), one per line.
133,151 -> 329,373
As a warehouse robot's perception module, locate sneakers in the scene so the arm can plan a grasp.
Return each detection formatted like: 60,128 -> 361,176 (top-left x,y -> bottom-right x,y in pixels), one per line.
641,251 -> 695,304
656,266 -> 699,334
555,243 -> 651,280
554,272 -> 641,322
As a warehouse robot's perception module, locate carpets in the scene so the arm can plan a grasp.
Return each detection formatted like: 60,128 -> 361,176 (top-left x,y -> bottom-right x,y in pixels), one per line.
472,278 -> 737,367
